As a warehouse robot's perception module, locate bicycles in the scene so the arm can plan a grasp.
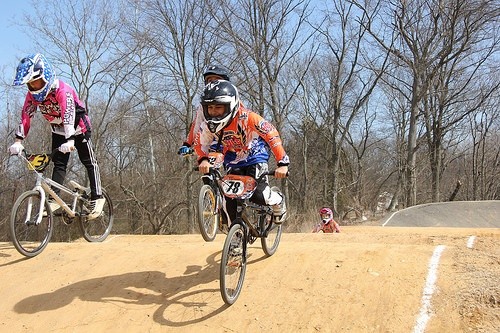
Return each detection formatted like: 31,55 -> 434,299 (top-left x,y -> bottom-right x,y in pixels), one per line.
178,148 -> 229,243
8,146 -> 114,256
194,166 -> 291,306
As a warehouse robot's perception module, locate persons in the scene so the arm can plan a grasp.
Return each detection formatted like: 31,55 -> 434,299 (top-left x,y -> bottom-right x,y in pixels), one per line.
311,207 -> 341,233
196,79 -> 290,266
177,65 -> 238,233
9,52 -> 106,219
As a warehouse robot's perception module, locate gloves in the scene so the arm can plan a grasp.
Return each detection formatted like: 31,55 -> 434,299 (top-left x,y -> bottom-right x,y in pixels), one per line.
178,144 -> 189,156
59,140 -> 74,152
10,142 -> 22,157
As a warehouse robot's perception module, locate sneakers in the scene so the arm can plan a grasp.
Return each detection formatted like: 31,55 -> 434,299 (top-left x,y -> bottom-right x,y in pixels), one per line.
88,196 -> 106,219
228,232 -> 242,256
43,198 -> 60,216
273,192 -> 287,225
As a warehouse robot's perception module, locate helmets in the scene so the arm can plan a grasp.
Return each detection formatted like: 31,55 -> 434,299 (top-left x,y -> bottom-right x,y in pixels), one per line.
200,80 -> 240,133
319,208 -> 334,224
11,53 -> 56,102
202,64 -> 229,84
